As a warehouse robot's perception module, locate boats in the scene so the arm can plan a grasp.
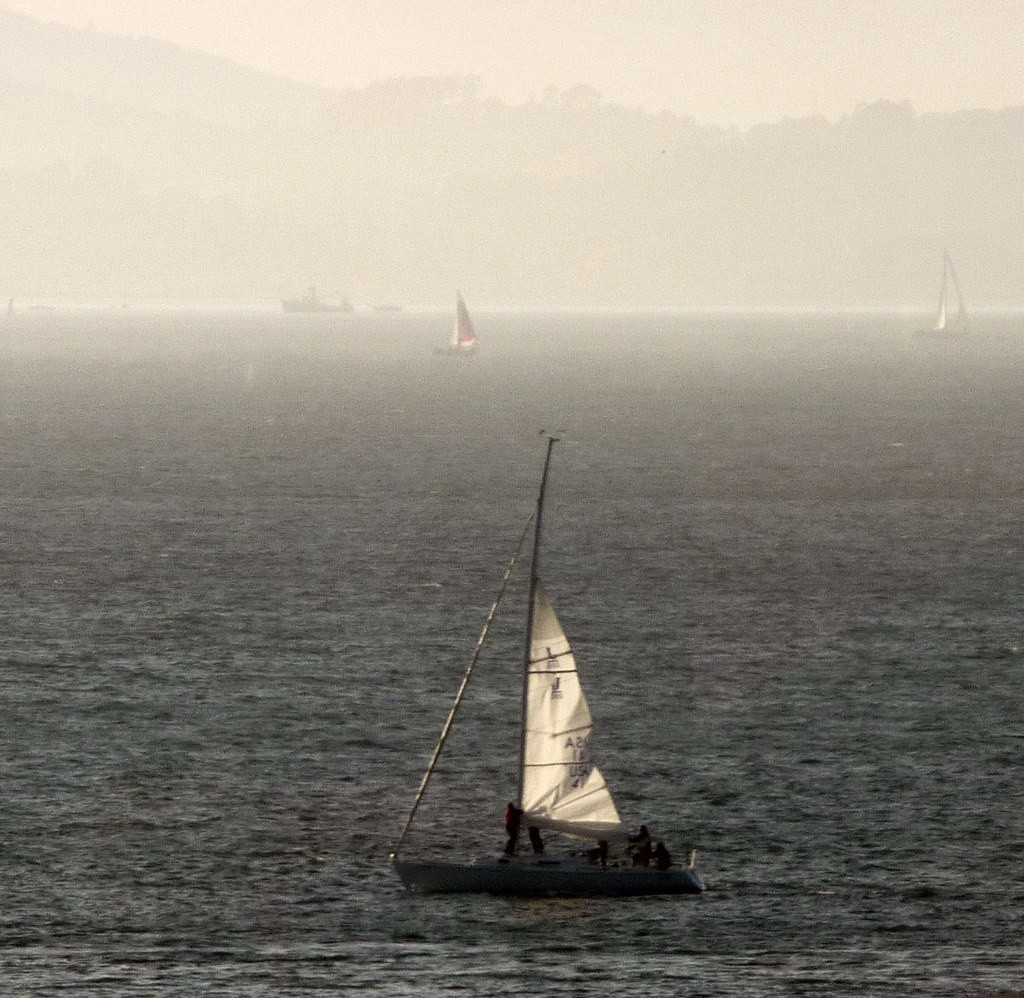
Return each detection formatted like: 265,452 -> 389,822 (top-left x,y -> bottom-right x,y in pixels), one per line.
287,291 -> 349,314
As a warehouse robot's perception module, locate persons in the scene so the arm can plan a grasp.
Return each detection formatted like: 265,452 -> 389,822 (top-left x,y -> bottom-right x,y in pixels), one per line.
528,823 -> 544,853
503,803 -> 524,856
625,825 -> 673,870
596,838 -> 610,867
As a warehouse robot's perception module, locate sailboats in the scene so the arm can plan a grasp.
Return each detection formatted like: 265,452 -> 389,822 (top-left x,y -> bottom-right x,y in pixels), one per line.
432,289 -> 481,358
922,248 -> 972,335
382,426 -> 705,898
